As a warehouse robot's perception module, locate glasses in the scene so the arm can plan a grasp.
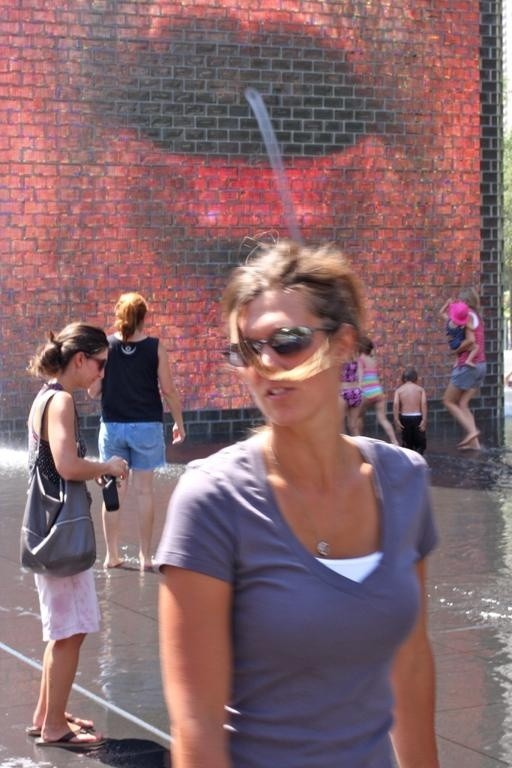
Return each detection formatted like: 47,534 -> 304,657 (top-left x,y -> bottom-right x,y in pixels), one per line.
86,356 -> 108,371
221,327 -> 336,367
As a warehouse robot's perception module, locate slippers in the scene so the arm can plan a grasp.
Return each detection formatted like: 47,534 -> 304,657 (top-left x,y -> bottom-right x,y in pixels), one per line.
34,727 -> 107,749
26,713 -> 93,736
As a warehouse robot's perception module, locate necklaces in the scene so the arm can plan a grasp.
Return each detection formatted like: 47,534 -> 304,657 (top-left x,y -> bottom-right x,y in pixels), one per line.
269,445 -> 370,557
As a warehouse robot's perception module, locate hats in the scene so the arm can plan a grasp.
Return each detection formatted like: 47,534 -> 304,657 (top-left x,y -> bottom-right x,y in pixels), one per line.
447,301 -> 470,326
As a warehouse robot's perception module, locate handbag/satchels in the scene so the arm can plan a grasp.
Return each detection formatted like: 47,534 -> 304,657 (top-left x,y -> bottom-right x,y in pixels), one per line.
20,461 -> 97,577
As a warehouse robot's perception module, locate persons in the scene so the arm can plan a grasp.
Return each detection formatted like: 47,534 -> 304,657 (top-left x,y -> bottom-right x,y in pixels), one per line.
338,288 -> 489,463
15,320 -> 133,752
85,289 -> 188,578
151,240 -> 446,767
